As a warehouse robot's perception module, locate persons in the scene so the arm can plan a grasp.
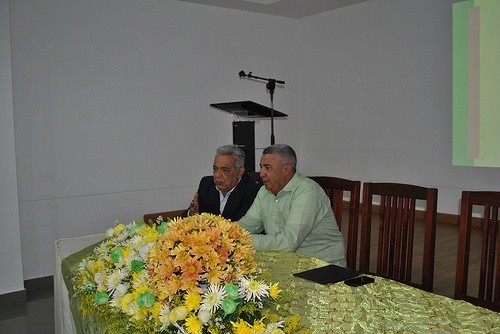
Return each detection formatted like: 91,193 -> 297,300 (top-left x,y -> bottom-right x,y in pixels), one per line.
234,144 -> 347,268
181,145 -> 264,222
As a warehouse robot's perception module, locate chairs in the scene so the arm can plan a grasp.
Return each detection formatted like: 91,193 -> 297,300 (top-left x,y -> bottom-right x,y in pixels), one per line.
306,176 -> 361,270
454,191 -> 500,311
359,182 -> 438,297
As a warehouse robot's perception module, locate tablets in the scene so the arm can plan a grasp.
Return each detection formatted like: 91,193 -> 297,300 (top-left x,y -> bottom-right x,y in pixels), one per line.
293,264 -> 361,285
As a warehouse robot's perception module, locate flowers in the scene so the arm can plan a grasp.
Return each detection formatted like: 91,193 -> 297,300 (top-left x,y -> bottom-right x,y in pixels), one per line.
68,212 -> 309,334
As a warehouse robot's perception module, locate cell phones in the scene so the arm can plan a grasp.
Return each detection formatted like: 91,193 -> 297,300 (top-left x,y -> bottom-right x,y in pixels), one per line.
344,276 -> 374,287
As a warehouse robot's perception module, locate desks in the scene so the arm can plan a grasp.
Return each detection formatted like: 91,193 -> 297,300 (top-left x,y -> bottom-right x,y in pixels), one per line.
53,234 -> 500,334
